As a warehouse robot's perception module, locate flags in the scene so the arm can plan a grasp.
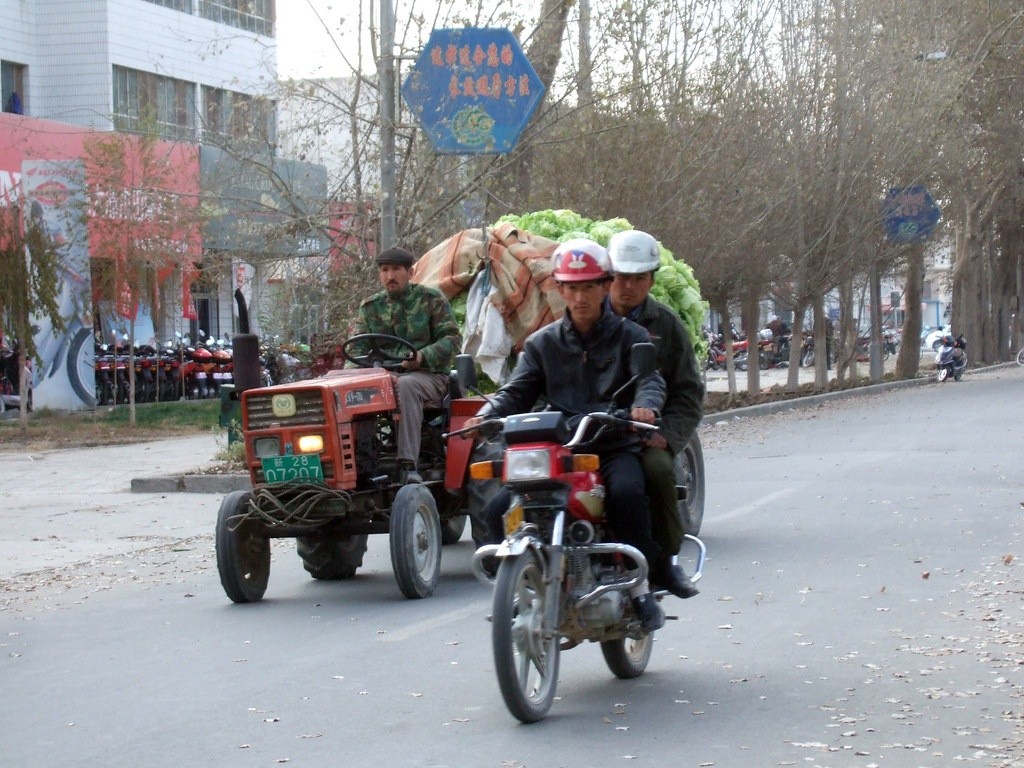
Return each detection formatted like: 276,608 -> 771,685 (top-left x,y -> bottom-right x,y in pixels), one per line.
113,247 -> 255,323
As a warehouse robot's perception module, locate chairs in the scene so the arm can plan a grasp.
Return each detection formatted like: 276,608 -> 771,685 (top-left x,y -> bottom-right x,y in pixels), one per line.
426,369 -> 461,427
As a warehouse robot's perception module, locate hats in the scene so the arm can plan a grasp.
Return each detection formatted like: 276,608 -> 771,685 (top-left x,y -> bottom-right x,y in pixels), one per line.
376,247 -> 414,268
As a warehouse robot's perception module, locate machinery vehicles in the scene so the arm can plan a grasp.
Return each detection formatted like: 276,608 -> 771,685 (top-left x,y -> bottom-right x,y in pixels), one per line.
211,288 -> 709,604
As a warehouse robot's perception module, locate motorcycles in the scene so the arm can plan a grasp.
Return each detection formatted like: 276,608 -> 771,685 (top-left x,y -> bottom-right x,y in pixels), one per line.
703,318 -> 901,372
442,354 -> 708,724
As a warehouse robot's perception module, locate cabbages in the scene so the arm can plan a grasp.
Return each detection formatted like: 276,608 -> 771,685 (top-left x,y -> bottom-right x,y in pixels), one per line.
450,209 -> 708,399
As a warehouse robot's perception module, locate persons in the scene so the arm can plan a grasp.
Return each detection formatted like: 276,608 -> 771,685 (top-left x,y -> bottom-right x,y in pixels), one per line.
606,229 -> 706,598
718,315 -> 833,370
344,247 -> 464,481
464,238 -> 665,631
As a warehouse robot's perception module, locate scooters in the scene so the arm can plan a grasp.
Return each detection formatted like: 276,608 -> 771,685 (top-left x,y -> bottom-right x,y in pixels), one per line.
935,334 -> 969,383
0,327 -> 302,413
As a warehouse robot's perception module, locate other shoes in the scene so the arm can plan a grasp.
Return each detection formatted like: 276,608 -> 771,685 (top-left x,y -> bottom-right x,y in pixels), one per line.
647,564 -> 700,598
629,592 -> 666,632
400,463 -> 422,483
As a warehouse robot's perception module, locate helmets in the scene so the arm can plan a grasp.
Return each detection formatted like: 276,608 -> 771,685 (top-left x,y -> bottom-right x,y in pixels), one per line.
551,238 -> 612,281
607,230 -> 662,274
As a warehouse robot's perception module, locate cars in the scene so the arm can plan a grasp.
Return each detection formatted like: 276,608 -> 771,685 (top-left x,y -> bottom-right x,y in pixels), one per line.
921,324 -> 952,352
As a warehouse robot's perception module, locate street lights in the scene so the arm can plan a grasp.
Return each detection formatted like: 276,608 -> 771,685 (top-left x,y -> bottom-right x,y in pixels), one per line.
860,50 -> 948,378
